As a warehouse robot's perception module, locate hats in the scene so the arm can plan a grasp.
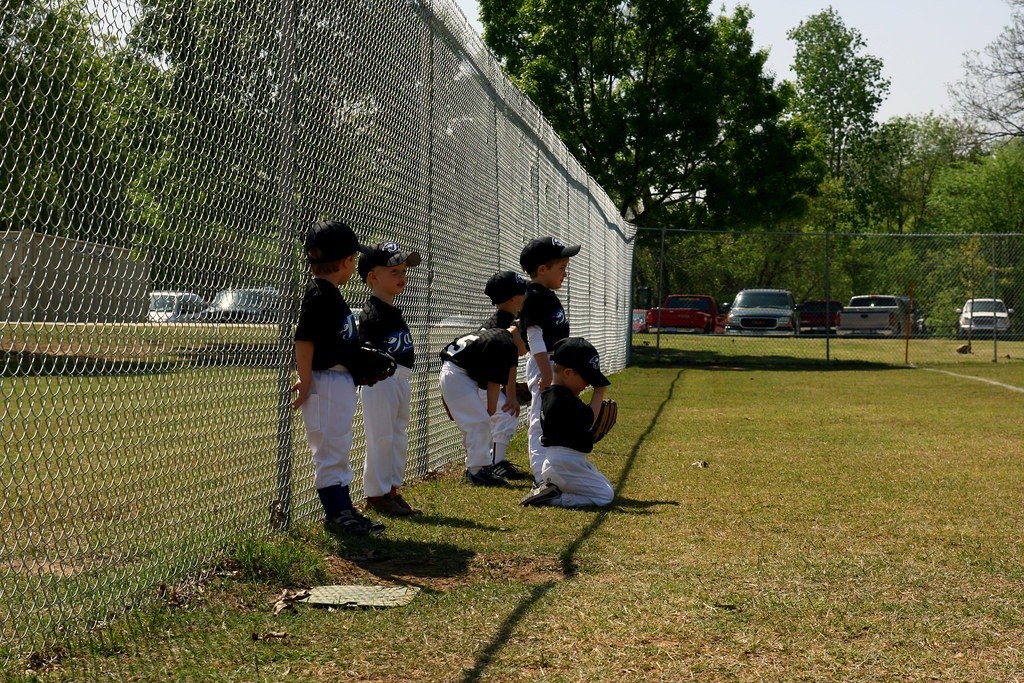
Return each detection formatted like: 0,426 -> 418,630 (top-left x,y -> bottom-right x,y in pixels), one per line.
550,336 -> 611,388
304,219 -> 373,262
358,240 -> 421,283
520,235 -> 582,274
484,271 -> 531,306
512,310 -> 521,327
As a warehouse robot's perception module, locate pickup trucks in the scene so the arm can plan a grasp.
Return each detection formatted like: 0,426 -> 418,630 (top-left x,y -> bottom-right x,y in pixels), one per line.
632,293 -> 727,334
798,294 -> 924,336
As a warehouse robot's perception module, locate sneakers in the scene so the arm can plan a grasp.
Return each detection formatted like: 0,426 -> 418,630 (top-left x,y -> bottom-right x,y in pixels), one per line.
365,496 -> 412,517
489,460 -> 529,479
393,495 -> 423,515
351,508 -> 385,534
533,480 -> 544,487
464,466 -> 508,487
518,482 -> 561,507
324,509 -> 367,536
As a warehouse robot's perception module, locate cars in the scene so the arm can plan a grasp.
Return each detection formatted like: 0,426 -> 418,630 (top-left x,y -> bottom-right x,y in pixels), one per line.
148,286 -> 293,326
956,299 -> 1015,333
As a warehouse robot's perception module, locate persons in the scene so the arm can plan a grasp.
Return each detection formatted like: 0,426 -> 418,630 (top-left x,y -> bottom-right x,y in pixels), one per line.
521,235 -> 581,490
478,271 -> 534,480
438,317 -> 529,491
518,336 -> 618,508
288,219 -> 388,539
353,240 -> 422,518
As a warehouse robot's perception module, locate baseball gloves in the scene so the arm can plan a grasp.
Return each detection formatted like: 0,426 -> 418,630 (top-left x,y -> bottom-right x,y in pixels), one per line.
346,341 -> 397,387
592,398 -> 618,444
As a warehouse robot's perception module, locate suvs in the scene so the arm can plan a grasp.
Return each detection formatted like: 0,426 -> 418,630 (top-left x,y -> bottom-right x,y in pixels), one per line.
722,289 -> 800,335
